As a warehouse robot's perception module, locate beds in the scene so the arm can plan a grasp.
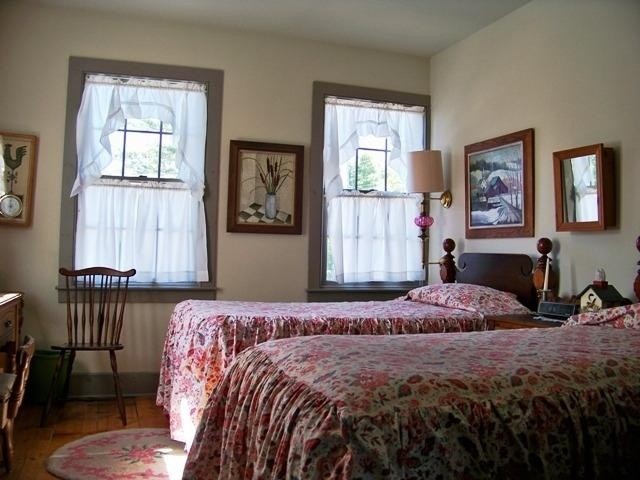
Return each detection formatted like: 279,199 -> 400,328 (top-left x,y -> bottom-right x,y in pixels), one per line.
155,238 -> 555,453
183,233 -> 639,480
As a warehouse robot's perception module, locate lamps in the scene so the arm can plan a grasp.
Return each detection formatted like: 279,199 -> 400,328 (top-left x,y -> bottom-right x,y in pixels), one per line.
411,149 -> 452,270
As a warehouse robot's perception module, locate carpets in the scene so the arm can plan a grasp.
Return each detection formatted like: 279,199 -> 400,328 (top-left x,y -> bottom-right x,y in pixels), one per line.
45,427 -> 189,480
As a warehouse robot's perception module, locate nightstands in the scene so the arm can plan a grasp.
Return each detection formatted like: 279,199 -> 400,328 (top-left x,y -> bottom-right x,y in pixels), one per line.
487,314 -> 562,331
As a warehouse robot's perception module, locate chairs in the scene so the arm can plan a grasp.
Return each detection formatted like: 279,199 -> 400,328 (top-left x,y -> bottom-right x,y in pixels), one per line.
41,267 -> 136,427
0,335 -> 35,457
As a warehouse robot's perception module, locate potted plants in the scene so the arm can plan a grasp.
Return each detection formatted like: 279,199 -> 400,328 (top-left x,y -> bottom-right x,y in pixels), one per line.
237,154 -> 294,219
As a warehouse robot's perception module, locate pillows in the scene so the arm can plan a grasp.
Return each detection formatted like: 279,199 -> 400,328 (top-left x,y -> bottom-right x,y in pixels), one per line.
561,301 -> 640,326
0,372 -> 17,396
398,282 -> 536,317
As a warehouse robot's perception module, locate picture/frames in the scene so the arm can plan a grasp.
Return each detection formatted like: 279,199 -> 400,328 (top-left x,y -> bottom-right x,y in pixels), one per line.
463,128 -> 535,240
0,130 -> 38,227
553,142 -> 618,232
226,140 -> 304,235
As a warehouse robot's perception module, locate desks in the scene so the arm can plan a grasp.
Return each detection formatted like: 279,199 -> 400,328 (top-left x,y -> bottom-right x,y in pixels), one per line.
0,291 -> 24,453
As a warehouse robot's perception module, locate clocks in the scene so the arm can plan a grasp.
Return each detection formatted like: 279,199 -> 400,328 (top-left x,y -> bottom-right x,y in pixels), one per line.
0,193 -> 24,219
